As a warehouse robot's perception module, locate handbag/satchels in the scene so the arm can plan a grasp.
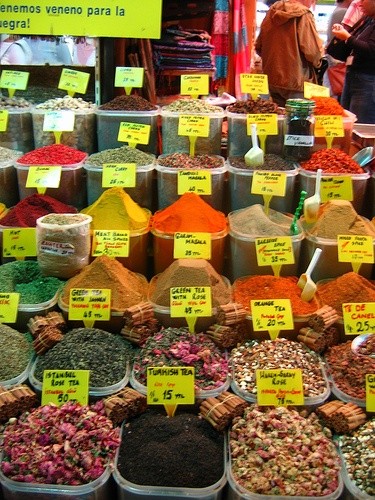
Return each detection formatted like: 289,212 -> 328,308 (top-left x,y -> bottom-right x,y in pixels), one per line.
328,62 -> 347,96
326,36 -> 352,62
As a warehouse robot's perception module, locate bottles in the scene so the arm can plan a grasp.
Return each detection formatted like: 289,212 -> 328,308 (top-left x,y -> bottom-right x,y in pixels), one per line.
283,99 -> 314,161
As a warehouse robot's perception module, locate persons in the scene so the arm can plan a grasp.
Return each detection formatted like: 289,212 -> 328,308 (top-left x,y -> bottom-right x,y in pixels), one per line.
254,0 -> 375,125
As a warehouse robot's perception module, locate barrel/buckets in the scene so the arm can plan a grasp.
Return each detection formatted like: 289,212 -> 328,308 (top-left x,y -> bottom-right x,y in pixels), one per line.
14,153 -> 88,211
89,208 -> 151,275
32,103 -> 96,155
83,153 -> 156,211
0,276 -> 375,500
0,103 -> 36,154
296,162 -> 370,214
226,105 -> 284,158
96,105 -> 160,159
150,210 -> 228,274
155,153 -> 226,211
312,110 -> 357,156
300,213 -> 375,284
228,209 -> 305,284
0,206 -> 78,267
0,149 -> 23,208
161,105 -> 226,156
227,156 -> 299,213
